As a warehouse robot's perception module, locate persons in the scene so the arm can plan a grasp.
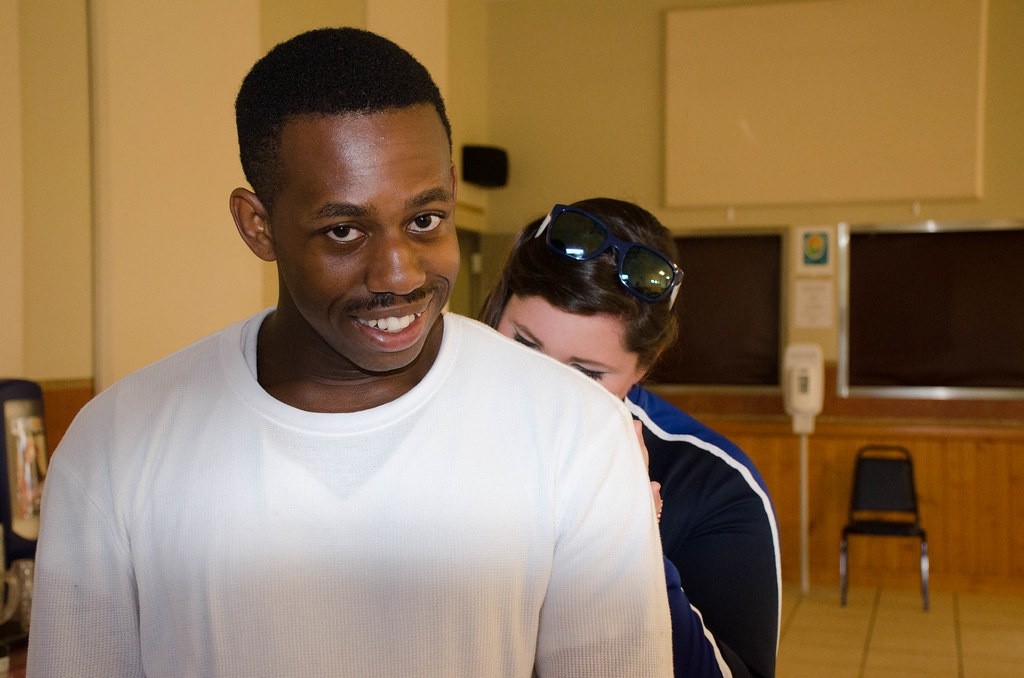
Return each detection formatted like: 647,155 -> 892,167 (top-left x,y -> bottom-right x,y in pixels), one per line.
23,28 -> 673,678
475,197 -> 783,678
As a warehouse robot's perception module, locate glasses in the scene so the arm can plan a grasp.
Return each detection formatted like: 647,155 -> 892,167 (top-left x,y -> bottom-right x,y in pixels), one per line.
534,203 -> 684,312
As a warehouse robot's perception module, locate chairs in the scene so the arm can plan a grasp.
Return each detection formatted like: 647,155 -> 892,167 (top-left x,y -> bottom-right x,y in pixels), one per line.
837,442 -> 933,611
